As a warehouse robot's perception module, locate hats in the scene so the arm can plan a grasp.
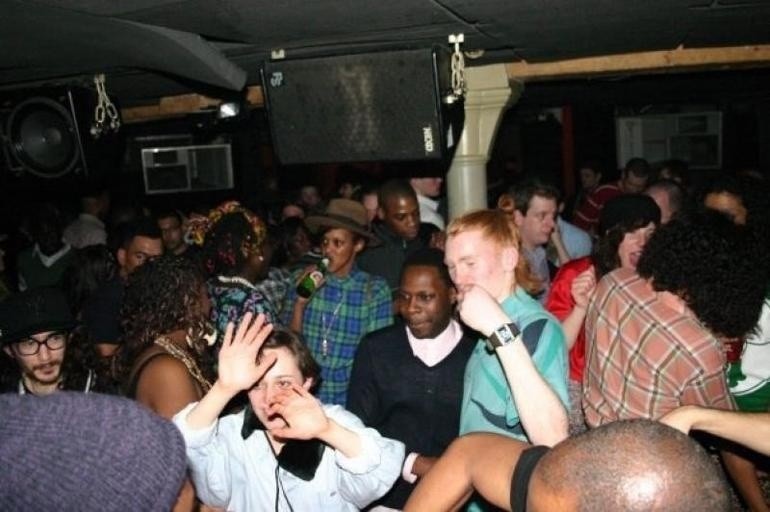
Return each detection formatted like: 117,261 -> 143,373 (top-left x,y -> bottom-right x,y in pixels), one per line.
305,198 -> 376,242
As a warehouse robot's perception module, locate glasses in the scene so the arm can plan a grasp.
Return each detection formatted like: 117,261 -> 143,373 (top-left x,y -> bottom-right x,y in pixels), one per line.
15,330 -> 68,356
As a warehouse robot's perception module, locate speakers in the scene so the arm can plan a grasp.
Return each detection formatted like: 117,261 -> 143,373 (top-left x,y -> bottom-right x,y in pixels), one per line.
257,42 -> 460,165
0,84 -> 126,202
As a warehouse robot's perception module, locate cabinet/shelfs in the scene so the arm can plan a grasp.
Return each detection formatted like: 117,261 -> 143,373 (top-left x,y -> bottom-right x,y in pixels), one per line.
615,110 -> 723,173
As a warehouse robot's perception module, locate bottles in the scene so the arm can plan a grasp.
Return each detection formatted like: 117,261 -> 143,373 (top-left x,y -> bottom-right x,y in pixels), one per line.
297,258 -> 331,296
